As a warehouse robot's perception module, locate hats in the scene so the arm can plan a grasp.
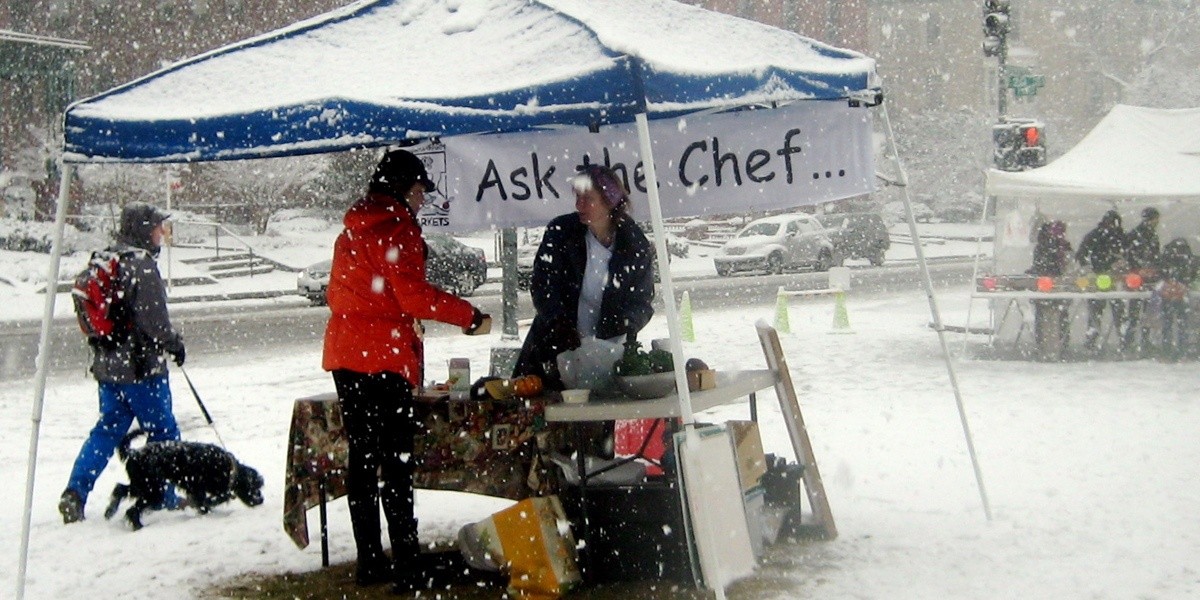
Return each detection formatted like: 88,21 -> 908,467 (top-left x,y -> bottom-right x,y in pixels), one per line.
379,148 -> 435,192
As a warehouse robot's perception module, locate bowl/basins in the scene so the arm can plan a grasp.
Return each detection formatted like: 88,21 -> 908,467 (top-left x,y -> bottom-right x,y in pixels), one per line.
561,389 -> 590,403
612,369 -> 676,400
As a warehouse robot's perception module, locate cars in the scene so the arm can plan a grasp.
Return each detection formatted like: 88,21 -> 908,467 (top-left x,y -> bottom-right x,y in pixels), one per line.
296,229 -> 487,308
811,211 -> 893,269
713,211 -> 836,275
516,244 -> 540,291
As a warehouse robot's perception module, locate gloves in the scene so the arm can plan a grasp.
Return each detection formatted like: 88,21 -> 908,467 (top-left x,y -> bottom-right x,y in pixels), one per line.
172,346 -> 186,366
551,321 -> 582,351
467,307 -> 486,334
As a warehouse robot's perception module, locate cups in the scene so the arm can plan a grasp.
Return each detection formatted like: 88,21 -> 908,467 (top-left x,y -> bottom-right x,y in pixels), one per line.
449,358 -> 471,400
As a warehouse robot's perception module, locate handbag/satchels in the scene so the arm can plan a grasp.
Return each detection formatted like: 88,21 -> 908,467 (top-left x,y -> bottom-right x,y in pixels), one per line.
476,495 -> 583,600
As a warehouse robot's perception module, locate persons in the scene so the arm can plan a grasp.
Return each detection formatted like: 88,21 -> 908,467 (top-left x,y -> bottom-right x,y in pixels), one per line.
322,150 -> 492,570
511,165 -> 657,501
1032,207 -> 1200,363
58,202 -> 186,527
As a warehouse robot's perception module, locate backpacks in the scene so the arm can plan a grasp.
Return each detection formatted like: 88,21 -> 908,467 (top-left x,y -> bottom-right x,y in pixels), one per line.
70,243 -> 150,346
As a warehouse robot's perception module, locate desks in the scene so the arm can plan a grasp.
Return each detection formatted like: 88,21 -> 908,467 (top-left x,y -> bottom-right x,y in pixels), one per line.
971,290 -> 1153,362
544,368 -> 781,599
284,389 -> 677,568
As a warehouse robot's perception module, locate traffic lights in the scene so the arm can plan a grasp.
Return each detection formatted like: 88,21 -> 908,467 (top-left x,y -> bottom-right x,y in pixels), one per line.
1019,123 -> 1046,169
981,0 -> 1009,57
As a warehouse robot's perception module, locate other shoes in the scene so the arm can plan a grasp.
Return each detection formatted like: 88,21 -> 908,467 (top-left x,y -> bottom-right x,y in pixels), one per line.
57,492 -> 85,523
393,570 -> 436,593
356,558 -> 392,585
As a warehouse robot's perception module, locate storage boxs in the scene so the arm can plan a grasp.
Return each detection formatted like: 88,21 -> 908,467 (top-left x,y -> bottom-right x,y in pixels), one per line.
557,471 -> 695,584
724,420 -> 803,559
687,369 -> 716,392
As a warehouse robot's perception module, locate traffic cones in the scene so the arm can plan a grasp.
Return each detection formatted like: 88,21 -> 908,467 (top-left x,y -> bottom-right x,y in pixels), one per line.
772,285 -> 792,341
825,289 -> 857,335
676,290 -> 695,344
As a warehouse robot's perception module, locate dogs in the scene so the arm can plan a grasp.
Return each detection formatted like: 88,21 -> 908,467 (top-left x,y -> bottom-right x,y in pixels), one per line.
106,430 -> 267,530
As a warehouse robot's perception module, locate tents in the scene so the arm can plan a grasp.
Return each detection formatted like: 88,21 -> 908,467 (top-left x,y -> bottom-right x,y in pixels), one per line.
15,0 -> 996,600
962,103 -> 1200,363
